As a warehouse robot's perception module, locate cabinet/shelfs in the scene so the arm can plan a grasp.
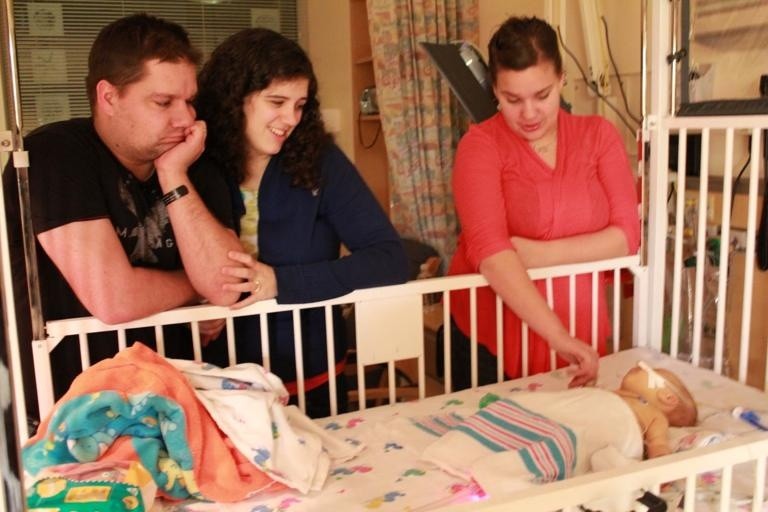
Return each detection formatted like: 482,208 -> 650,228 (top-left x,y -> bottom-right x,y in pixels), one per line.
346,1 -> 392,222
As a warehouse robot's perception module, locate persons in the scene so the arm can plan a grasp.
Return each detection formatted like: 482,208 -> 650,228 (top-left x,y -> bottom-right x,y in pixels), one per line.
444,15 -> 642,387
568,360 -> 699,459
2,14 -> 246,483
196,28 -> 409,416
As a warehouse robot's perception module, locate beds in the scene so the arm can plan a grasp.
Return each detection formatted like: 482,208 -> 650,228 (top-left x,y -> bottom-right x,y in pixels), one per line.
1,114 -> 768,512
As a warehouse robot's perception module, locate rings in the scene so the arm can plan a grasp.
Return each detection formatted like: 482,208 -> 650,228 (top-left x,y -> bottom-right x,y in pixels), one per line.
254,280 -> 260,291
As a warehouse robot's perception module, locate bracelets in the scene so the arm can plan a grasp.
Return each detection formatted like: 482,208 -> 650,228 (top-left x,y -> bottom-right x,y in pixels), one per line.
159,185 -> 192,207
